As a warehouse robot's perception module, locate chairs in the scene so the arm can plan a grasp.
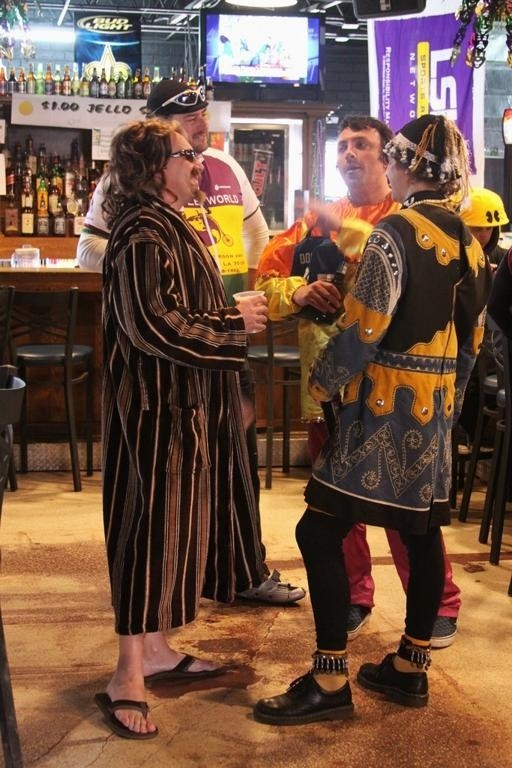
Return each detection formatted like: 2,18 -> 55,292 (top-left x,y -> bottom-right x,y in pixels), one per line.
489,389 -> 512,565
0,285 -> 19,492
9,287 -> 94,492
459,312 -> 508,544
247,313 -> 301,489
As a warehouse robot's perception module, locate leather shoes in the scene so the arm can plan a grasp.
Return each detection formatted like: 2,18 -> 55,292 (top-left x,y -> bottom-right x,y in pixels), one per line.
254,673 -> 354,726
357,653 -> 428,705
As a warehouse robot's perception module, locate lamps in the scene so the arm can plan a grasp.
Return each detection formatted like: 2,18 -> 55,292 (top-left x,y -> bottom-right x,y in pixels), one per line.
501,95 -> 512,235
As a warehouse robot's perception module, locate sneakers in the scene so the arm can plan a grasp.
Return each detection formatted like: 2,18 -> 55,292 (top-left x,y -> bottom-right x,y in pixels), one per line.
347,604 -> 371,641
235,579 -> 306,606
431,616 -> 456,647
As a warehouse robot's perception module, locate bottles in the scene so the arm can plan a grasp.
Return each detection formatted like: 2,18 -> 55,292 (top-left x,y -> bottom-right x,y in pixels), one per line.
21,191 -> 34,236
22,175 -> 35,209
37,194 -> 49,236
126,68 -> 134,99
49,137 -> 101,237
151,66 -> 160,88
26,63 -> 36,94
231,142 -> 281,228
133,68 -> 142,98
72,63 -> 80,96
53,64 -> 62,94
45,64 -> 54,96
4,196 -> 21,236
6,157 -> 16,194
79,72 -> 90,96
63,65 -> 71,96
10,244 -> 40,268
170,66 -> 178,83
207,76 -> 214,101
37,176 -> 48,210
35,63 -> 45,94
0,67 -> 7,96
108,66 -> 117,98
98,67 -> 108,97
37,143 -> 50,178
178,66 -> 186,85
313,272 -> 345,322
18,67 -> 27,94
142,67 -> 152,98
197,66 -> 205,91
116,70 -> 126,98
187,76 -> 195,89
24,139 -> 37,179
7,67 -> 17,95
13,145 -> 24,193
90,68 -> 99,98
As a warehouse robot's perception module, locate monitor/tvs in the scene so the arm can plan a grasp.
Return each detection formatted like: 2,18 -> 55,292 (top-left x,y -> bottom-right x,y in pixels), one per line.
198,7 -> 328,103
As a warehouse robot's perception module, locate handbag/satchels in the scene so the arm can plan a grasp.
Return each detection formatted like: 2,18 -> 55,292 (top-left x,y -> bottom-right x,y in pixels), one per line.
290,225 -> 348,325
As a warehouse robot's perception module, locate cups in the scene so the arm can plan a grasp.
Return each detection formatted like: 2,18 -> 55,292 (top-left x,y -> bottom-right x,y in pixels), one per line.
232,290 -> 266,333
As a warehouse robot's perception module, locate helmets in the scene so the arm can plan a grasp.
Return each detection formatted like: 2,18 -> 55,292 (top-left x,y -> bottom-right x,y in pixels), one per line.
459,188 -> 510,227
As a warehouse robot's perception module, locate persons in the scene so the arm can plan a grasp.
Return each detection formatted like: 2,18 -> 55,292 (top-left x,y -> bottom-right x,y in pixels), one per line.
254,115 -> 462,648
454,189 -> 511,447
94,120 -> 269,740
76,79 -> 306,607
254,114 -> 493,726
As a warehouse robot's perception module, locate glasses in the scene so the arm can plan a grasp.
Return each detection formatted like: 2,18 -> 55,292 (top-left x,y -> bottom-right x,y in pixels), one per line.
167,149 -> 197,162
147,86 -> 207,119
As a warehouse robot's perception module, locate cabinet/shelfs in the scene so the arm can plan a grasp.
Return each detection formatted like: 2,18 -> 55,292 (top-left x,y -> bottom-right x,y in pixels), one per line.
0,95 -> 342,260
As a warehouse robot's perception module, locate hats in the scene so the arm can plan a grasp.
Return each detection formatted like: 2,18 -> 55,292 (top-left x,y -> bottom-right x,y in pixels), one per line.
382,115 -> 462,183
147,78 -> 208,115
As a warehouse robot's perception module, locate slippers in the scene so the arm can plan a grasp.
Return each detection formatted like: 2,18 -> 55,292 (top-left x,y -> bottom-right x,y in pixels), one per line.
144,652 -> 227,682
94,692 -> 158,740
